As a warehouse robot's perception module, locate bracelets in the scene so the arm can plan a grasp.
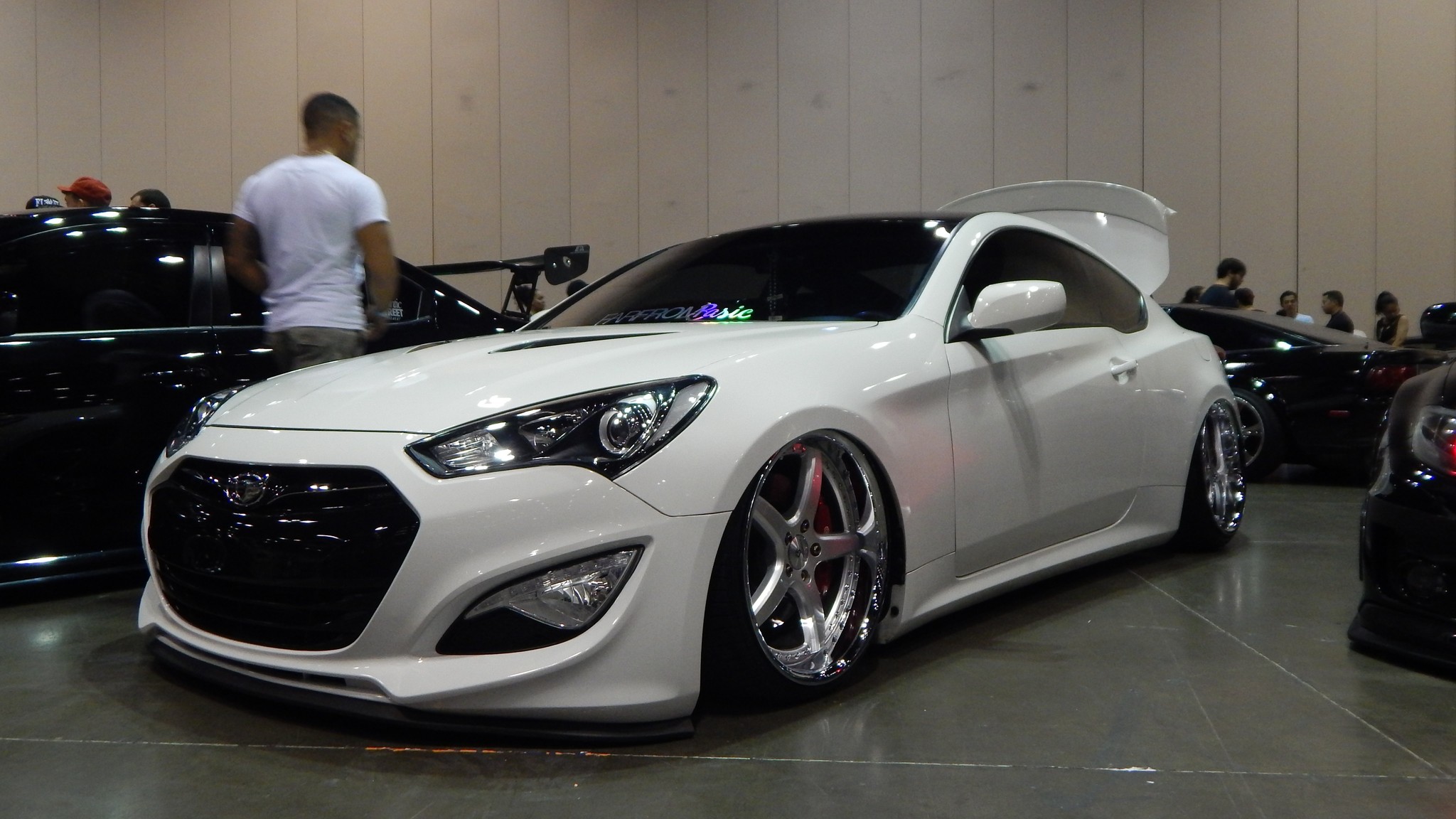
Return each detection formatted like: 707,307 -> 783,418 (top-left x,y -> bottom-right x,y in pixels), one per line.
371,304 -> 394,321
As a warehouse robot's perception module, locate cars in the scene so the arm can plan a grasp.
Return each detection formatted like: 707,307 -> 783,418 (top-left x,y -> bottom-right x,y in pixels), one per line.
1157,302 -> 1454,484
1347,300 -> 1456,679
0,195 -> 596,588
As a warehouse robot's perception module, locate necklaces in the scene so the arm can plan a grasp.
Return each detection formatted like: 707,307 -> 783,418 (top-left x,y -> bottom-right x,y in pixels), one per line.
301,148 -> 334,156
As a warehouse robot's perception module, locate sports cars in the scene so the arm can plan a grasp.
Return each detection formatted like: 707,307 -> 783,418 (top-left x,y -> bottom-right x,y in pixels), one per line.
132,178 -> 1250,741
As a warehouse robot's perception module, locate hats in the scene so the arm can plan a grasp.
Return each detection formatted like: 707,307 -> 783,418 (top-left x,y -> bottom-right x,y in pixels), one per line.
57,176 -> 112,207
25,195 -> 63,208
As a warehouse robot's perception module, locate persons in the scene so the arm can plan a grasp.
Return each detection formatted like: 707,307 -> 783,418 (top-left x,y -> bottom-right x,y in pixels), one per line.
1374,291 -> 1410,350
1275,290 -> 1315,325
1320,290 -> 1354,334
1178,285 -> 1207,306
128,188 -> 171,210
1234,288 -> 1266,312
223,92 -> 398,373
1197,257 -> 1247,310
55,177 -> 111,210
24,195 -> 64,211
